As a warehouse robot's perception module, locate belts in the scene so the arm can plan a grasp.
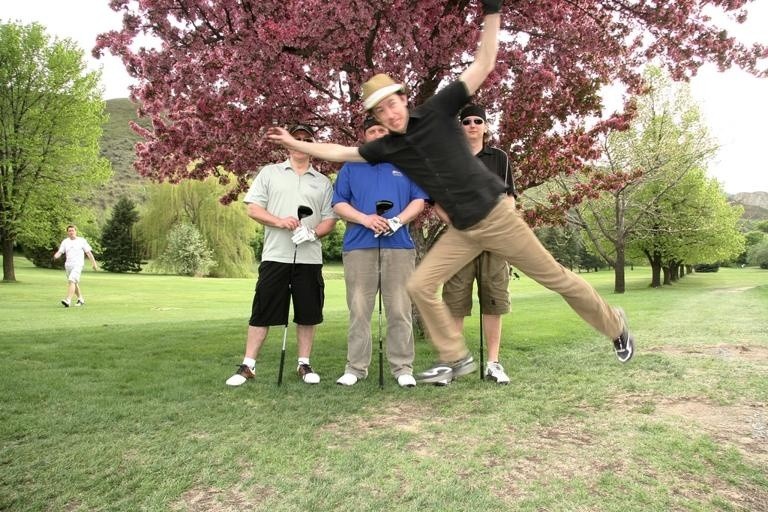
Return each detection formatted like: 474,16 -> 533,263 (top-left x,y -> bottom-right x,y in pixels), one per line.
490,192 -> 506,209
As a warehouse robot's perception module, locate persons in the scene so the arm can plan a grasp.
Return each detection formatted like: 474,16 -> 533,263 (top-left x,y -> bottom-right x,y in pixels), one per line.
53,225 -> 99,308
329,114 -> 433,388
263,0 -> 639,386
436,102 -> 516,386
225,123 -> 343,388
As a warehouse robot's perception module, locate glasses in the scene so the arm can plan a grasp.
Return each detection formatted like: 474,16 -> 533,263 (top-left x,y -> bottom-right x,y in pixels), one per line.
462,120 -> 483,124
296,136 -> 314,142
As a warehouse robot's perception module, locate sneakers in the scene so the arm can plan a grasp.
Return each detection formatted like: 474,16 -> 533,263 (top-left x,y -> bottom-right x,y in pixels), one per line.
224,363 -> 257,386
417,353 -> 478,383
484,362 -> 509,384
75,299 -> 85,307
337,369 -> 369,385
611,307 -> 634,362
296,361 -> 320,384
61,298 -> 71,308
398,374 -> 416,388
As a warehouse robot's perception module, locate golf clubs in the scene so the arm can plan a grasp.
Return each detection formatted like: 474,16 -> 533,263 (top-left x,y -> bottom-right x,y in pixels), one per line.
376,200 -> 395,394
278,205 -> 313,385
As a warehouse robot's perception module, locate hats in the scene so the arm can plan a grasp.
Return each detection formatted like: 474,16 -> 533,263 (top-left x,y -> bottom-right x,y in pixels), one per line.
460,106 -> 488,120
288,123 -> 314,136
361,114 -> 381,133
358,74 -> 405,113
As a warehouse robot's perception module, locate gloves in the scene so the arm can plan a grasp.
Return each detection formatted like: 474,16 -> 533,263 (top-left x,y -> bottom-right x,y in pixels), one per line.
291,226 -> 318,245
373,217 -> 402,239
